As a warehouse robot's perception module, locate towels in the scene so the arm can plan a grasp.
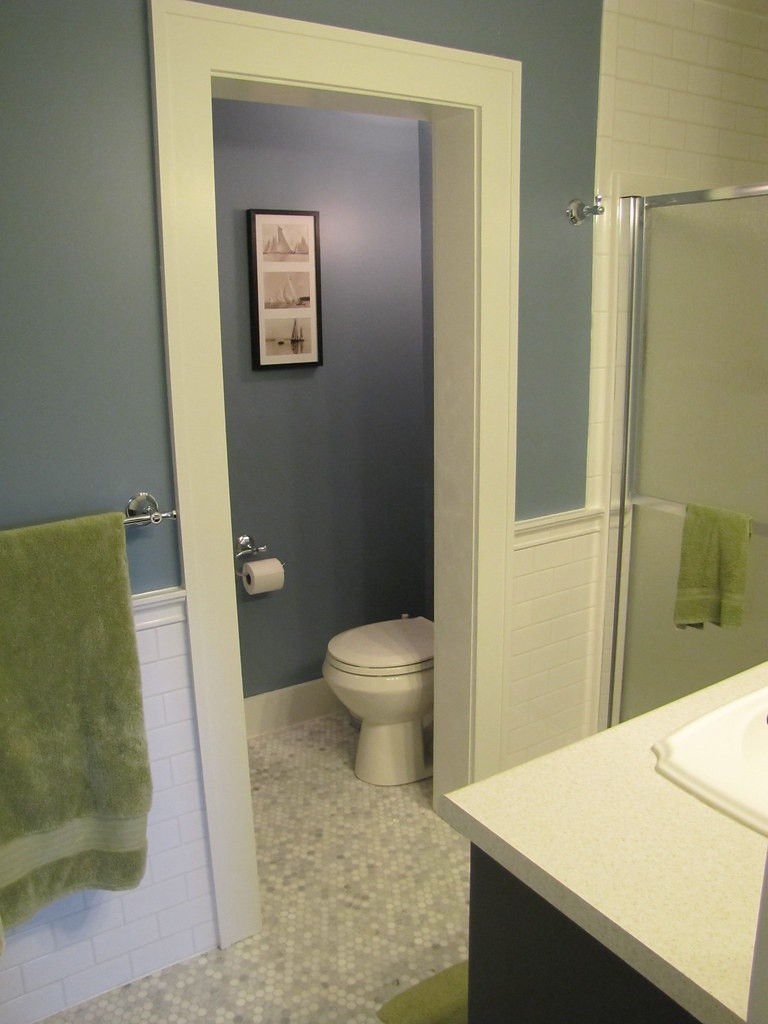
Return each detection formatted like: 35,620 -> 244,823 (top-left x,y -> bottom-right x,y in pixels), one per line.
2,511 -> 155,931
673,502 -> 754,635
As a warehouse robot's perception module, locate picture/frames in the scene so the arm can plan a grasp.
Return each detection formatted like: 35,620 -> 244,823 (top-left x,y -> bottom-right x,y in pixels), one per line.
245,207 -> 322,371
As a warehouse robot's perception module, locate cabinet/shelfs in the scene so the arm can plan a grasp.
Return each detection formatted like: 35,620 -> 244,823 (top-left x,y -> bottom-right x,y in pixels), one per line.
469,841 -> 699,1023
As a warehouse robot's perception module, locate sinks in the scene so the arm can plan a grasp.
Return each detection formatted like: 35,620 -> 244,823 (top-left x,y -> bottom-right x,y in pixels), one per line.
651,684 -> 767,840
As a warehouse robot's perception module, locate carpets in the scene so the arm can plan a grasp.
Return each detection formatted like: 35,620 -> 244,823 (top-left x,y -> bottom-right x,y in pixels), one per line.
374,957 -> 471,1024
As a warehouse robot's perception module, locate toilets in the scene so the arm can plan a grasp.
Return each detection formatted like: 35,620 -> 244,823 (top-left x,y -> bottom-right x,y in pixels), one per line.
321,617 -> 435,786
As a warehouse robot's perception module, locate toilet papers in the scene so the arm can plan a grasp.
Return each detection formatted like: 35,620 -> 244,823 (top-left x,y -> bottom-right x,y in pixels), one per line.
242,557 -> 285,596
